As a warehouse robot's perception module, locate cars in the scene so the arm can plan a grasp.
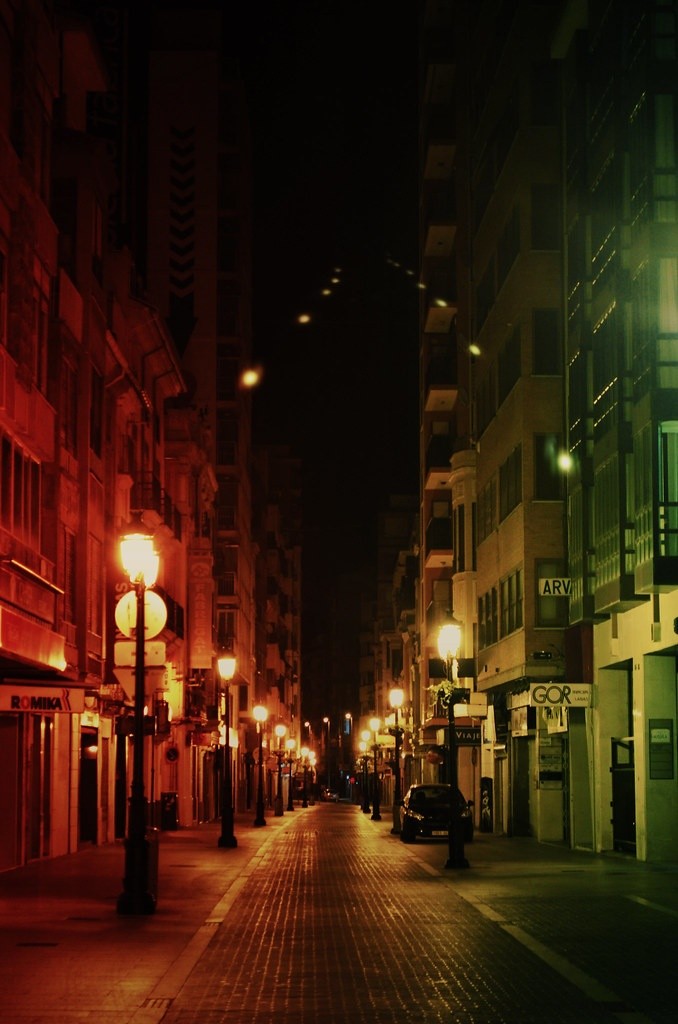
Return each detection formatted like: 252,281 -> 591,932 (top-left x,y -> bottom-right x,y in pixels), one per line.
400,784 -> 475,846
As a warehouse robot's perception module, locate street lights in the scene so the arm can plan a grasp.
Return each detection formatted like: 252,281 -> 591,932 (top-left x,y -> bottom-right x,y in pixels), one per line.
370,718 -> 381,821
358,730 -> 372,814
308,759 -> 317,806
275,725 -> 287,816
286,739 -> 295,811
218,649 -> 237,848
390,688 -> 404,834
115,511 -> 155,917
251,705 -> 269,826
437,624 -> 471,871
301,746 -> 309,808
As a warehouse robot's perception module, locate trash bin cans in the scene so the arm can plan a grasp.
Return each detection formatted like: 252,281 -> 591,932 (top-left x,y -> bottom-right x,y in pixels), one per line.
160,792 -> 178,831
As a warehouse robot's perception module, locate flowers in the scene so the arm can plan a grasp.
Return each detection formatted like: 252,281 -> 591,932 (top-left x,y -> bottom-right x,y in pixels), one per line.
422,677 -> 462,709
